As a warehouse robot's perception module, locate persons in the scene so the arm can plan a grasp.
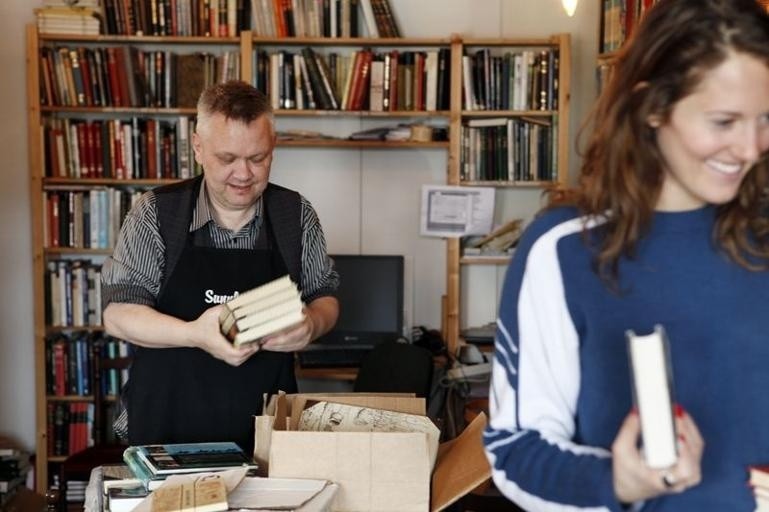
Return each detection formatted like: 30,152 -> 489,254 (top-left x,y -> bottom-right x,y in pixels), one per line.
101,82 -> 339,461
482,0 -> 769,512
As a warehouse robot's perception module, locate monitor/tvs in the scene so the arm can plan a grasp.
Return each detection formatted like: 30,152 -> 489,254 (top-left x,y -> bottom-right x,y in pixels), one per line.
310,254 -> 405,345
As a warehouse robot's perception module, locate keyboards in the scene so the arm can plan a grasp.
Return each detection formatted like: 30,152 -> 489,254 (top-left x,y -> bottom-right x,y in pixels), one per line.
297,349 -> 371,367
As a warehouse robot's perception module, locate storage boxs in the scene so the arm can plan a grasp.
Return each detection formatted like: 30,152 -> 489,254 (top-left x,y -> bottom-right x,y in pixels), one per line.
253,390 -> 494,512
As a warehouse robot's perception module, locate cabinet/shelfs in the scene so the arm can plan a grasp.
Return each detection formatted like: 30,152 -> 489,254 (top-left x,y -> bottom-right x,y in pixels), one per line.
596,2 -> 663,99
25,19 -> 573,501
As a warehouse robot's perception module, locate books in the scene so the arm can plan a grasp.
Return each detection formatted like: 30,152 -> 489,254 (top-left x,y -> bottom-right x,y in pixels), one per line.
249,43 -> 450,111
217,274 -> 306,347
43,259 -> 103,325
46,466 -> 90,503
626,324 -> 679,470
42,188 -> 146,251
459,113 -> 554,182
103,440 -> 249,512
46,400 -> 109,457
462,217 -> 525,256
463,46 -> 558,110
39,39 -> 243,107
43,330 -> 131,397
33,0 -> 404,37
42,111 -> 201,180
